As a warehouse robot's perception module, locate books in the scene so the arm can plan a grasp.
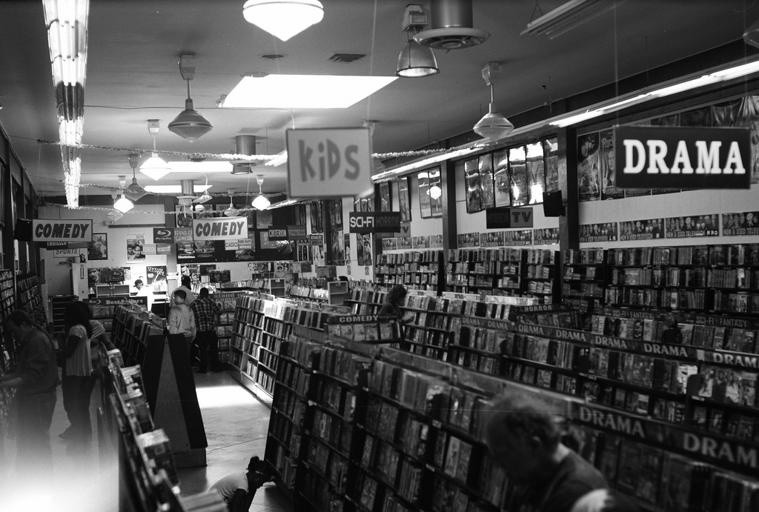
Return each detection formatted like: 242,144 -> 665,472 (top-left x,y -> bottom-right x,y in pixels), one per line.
1,244 -> 759,512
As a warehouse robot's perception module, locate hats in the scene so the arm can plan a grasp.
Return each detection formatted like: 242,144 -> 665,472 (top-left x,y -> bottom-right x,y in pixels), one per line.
248,456 -> 282,480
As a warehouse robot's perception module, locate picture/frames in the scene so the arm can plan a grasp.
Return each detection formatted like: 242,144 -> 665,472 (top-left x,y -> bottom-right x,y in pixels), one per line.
374,135 -> 557,221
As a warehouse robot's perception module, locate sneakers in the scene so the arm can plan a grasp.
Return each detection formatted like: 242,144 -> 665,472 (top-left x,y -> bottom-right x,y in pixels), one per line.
196,369 -> 206,373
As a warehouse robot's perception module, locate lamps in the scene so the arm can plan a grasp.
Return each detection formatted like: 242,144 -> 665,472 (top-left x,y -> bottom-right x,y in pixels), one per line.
396,1 -> 440,78
473,61 -> 515,143
242,1 -> 325,42
413,1 -> 491,50
519,1 -> 622,41
108,120 -> 270,222
168,52 -> 213,143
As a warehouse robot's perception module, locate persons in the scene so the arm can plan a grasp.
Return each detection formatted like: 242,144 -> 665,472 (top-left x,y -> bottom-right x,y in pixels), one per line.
607,146 -> 616,185
